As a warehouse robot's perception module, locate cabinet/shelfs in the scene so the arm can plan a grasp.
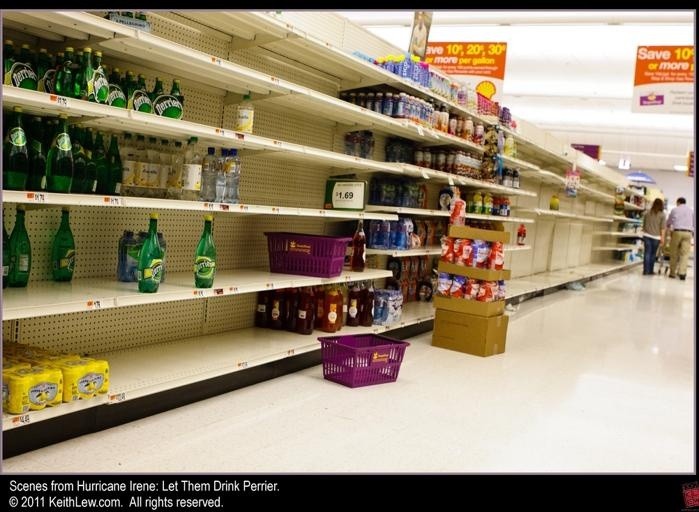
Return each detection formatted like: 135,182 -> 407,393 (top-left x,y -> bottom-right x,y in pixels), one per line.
1,13 -> 664,433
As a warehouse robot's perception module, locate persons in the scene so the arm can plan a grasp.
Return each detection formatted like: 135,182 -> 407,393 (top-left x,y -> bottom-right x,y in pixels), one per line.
640,198 -> 666,275
665,196 -> 694,281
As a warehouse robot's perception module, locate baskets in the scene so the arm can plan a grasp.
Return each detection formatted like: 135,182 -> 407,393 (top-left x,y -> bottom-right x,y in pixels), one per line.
264,231 -> 352,278
317,333 -> 410,388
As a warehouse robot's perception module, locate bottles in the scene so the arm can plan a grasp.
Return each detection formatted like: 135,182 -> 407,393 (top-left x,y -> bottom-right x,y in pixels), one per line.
8,203 -> 32,286
52,207 -> 77,282
115,229 -> 169,283
2,208 -> 11,288
2,106 -> 242,204
3,36 -> 185,117
236,94 -> 255,135
249,157 -> 526,338
338,54 -> 518,158
191,217 -> 217,288
136,214 -> 162,293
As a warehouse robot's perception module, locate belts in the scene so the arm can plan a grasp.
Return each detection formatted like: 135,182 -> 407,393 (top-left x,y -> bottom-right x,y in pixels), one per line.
675,229 -> 687,231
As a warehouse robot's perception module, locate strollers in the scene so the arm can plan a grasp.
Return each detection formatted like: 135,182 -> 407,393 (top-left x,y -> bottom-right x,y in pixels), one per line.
656,229 -> 676,275
